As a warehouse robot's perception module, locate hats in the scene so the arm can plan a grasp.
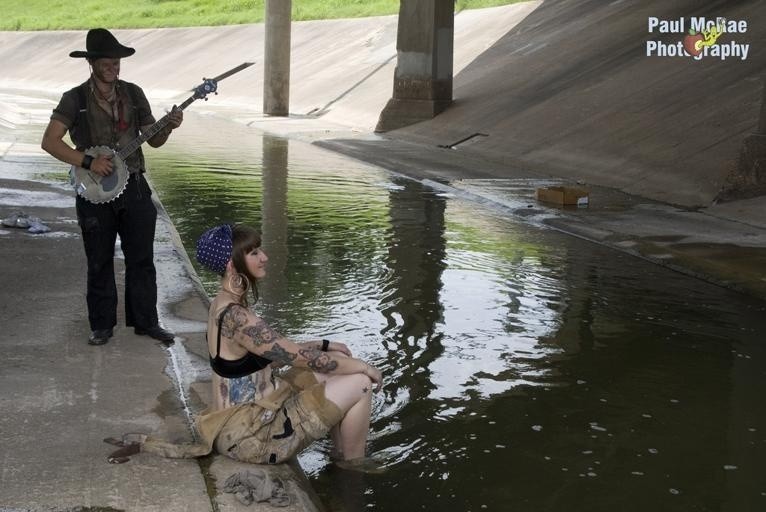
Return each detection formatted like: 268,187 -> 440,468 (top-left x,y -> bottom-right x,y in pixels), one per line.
196,225 -> 233,277
68,27 -> 136,60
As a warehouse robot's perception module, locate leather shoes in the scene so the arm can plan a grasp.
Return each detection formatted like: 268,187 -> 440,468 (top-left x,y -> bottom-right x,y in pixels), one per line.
134,326 -> 176,342
88,328 -> 114,346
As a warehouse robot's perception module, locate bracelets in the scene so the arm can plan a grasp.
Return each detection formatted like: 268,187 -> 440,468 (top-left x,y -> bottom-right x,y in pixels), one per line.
322,340 -> 329,351
81,154 -> 94,171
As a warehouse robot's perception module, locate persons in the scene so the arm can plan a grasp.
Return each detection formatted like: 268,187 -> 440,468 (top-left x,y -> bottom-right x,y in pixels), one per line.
194,224 -> 383,466
41,27 -> 186,346
